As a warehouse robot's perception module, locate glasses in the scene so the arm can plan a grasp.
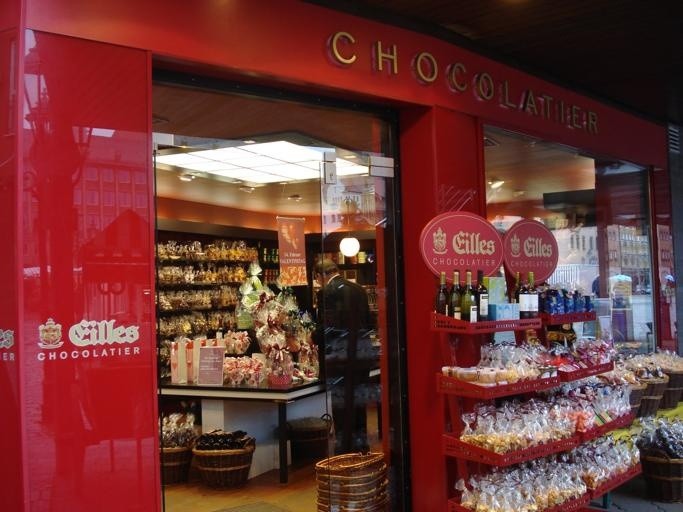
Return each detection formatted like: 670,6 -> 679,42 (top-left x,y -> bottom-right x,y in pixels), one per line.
316,278 -> 319,283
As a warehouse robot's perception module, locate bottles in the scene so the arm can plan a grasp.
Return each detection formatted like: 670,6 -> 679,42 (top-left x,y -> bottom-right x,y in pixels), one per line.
433,268 -> 539,323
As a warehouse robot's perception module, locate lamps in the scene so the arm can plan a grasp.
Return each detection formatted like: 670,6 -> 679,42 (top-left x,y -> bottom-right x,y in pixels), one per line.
339,197 -> 359,259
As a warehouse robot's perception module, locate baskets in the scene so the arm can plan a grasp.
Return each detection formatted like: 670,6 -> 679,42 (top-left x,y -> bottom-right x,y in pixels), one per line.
315,452 -> 393,512
630,381 -> 648,416
640,447 -> 683,503
192,437 -> 255,488
659,371 -> 683,409
160,439 -> 195,485
637,372 -> 670,417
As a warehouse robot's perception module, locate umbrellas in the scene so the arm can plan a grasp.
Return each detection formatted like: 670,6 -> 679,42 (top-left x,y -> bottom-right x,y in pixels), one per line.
665,275 -> 674,282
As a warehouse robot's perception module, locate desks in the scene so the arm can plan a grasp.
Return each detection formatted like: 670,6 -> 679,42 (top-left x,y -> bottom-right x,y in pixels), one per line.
157,371 -> 383,484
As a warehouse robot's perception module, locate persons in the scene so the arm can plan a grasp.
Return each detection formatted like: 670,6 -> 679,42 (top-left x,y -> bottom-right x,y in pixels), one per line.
306,259 -> 375,457
666,279 -> 673,288
592,275 -> 600,298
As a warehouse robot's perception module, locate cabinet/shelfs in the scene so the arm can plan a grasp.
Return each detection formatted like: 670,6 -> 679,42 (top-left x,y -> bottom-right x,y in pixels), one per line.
518,310 -> 643,510
430,304 -> 590,512
154,254 -> 262,369
